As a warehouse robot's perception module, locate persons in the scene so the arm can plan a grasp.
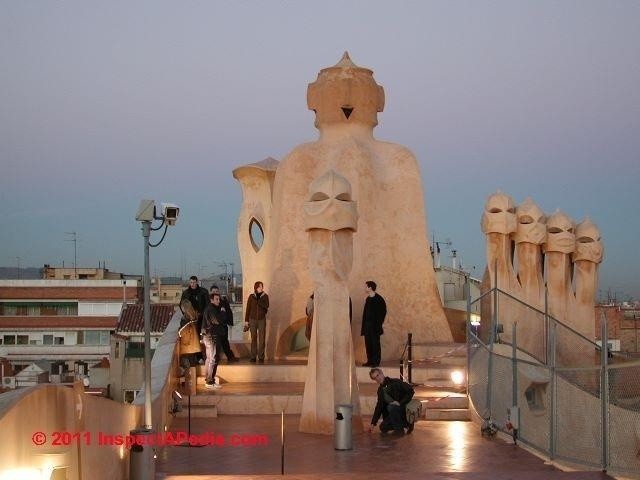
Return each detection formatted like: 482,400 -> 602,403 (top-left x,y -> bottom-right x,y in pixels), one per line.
360,280 -> 387,366
178,276 -> 239,390
244,281 -> 269,363
368,368 -> 414,434
349,297 -> 352,324
305,293 -> 314,342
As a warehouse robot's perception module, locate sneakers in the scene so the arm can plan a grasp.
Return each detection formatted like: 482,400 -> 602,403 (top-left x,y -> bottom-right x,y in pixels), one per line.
205,382 -> 222,389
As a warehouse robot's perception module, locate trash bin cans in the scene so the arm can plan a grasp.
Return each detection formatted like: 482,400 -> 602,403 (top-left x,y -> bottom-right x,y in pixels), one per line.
334,405 -> 353,450
130,428 -> 155,480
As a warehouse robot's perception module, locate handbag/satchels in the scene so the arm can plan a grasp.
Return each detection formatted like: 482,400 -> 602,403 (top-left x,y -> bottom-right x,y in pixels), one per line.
406,399 -> 422,424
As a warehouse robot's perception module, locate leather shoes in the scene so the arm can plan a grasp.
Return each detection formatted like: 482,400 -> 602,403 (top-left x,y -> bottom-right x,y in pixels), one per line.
392,422 -> 414,434
228,357 -> 240,363
250,358 -> 264,363
362,361 -> 380,367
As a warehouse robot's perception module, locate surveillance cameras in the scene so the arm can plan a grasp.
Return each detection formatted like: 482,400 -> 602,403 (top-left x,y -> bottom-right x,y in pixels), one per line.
160,202 -> 179,227
